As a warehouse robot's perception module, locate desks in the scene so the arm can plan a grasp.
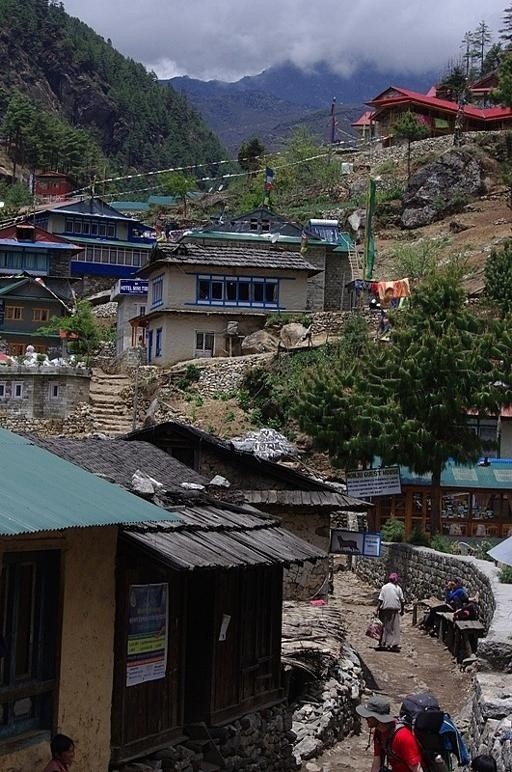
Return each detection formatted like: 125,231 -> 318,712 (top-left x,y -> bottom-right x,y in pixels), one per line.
411,598 -> 485,660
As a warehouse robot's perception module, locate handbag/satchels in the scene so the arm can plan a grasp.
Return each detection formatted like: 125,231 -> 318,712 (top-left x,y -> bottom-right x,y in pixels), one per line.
365,617 -> 384,640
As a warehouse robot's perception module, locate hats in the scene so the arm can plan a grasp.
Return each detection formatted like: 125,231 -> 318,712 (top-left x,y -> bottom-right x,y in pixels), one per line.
356,696 -> 397,724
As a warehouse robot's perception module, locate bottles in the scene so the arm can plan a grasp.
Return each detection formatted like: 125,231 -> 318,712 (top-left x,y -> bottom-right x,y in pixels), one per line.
433,752 -> 443,762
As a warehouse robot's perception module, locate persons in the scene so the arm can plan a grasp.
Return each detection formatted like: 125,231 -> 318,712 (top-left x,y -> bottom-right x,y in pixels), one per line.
138,335 -> 143,344
471,755 -> 497,771
416,577 -> 465,632
373,572 -> 405,653
355,696 -> 430,771
379,287 -> 395,333
43,734 -> 75,772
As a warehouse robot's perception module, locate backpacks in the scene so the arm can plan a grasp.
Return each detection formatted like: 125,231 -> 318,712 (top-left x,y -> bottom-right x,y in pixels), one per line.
453,601 -> 478,621
375,692 -> 471,772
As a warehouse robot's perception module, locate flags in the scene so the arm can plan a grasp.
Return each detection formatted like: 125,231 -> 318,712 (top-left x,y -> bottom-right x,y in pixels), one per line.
263,166 -> 274,209
